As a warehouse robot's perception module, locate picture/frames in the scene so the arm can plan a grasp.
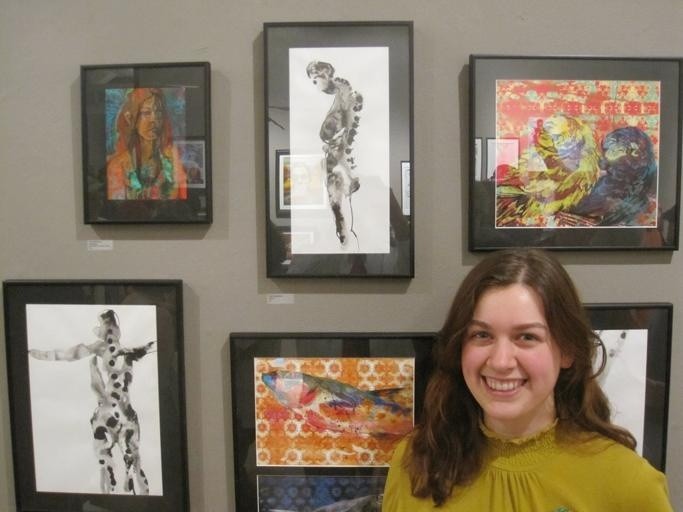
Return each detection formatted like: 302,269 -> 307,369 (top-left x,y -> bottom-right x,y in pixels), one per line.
262,21 -> 416,280
79,60 -> 212,227
228,331 -> 435,512
0,279 -> 189,512
577,300 -> 674,473
467,54 -> 683,253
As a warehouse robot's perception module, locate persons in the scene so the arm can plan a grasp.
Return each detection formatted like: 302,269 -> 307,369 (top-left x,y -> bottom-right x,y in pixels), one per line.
381,248 -> 672,512
109,87 -> 189,204
